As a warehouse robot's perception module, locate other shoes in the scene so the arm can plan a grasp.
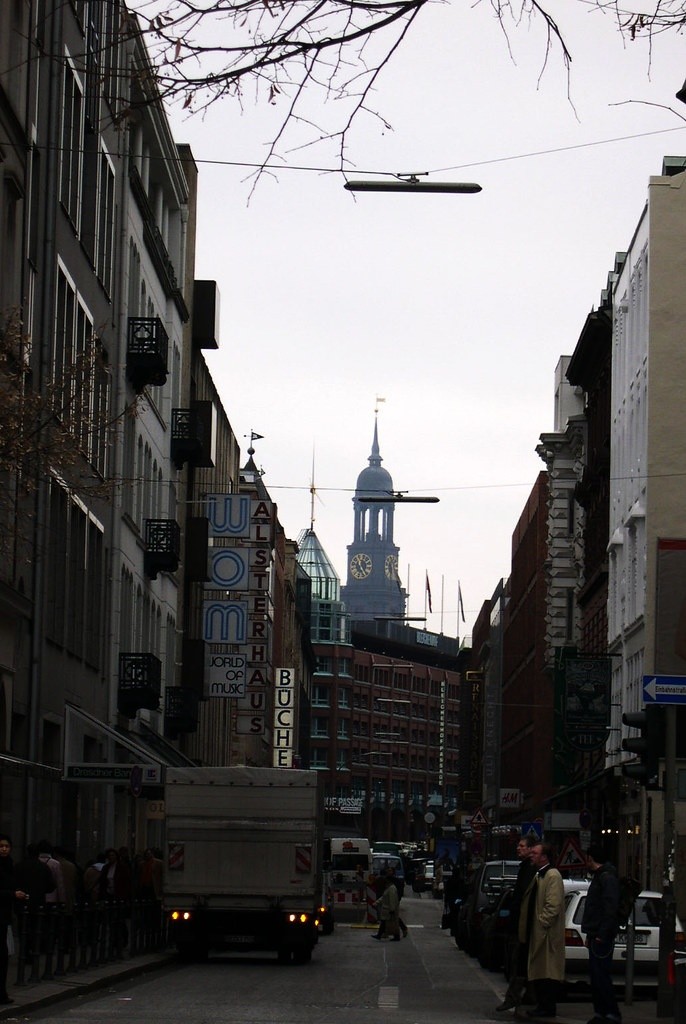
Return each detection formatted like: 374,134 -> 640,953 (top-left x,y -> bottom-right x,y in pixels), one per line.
586,1014 -> 619,1024
401,926 -> 407,937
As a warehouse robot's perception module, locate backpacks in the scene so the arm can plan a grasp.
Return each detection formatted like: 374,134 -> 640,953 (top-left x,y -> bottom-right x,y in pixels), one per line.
611,875 -> 644,927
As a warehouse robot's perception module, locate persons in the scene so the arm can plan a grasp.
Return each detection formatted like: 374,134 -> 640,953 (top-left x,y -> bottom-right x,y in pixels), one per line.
497,836 -> 535,1009
517,842 -> 564,1016
0,835 -> 14,959
364,852 -> 447,943
581,846 -> 623,1024
14,835 -> 172,966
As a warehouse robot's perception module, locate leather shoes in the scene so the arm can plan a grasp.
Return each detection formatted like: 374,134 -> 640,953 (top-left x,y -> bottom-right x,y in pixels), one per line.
526,1002 -> 557,1017
370,933 -> 382,940
495,993 -> 521,1011
389,935 -> 400,942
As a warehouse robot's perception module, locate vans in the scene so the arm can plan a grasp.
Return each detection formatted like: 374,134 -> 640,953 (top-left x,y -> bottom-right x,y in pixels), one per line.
329,837 -> 374,921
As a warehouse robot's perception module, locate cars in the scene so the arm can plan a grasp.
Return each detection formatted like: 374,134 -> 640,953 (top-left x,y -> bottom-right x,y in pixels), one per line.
369,844 -> 522,937
540,889 -> 685,1000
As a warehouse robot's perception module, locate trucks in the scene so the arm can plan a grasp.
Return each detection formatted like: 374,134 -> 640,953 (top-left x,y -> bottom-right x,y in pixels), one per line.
164,764 -> 328,968
